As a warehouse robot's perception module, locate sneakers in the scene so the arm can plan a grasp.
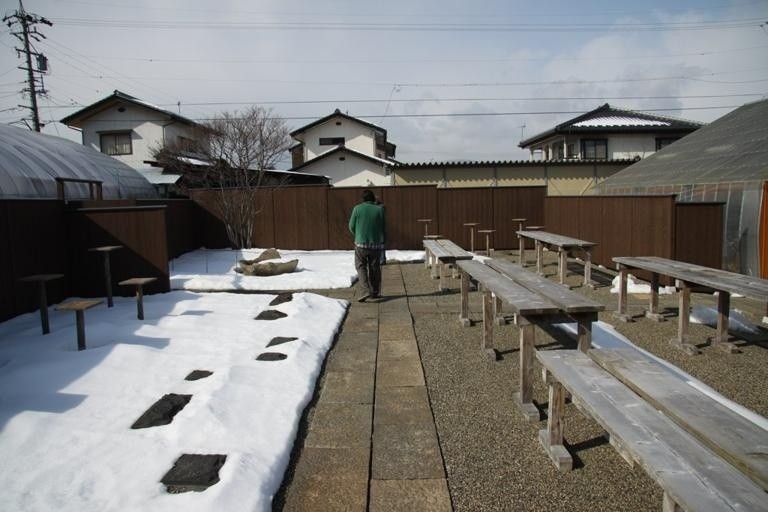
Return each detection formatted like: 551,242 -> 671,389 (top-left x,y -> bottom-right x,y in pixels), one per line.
357,292 -> 384,301
379,260 -> 386,265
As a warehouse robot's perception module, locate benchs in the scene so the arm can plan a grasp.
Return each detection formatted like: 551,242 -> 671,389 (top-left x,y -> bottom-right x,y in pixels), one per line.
453,258 -> 606,415
518,230 -> 597,290
535,347 -> 768,512
612,257 -> 768,357
424,239 -> 478,294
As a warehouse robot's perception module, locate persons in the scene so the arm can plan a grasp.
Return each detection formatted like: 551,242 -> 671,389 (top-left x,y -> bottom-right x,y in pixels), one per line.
373,194 -> 385,265
349,190 -> 385,301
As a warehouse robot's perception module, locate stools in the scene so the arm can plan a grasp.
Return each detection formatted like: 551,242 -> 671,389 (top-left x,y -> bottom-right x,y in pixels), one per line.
478,230 -> 497,256
425,235 -> 445,239
